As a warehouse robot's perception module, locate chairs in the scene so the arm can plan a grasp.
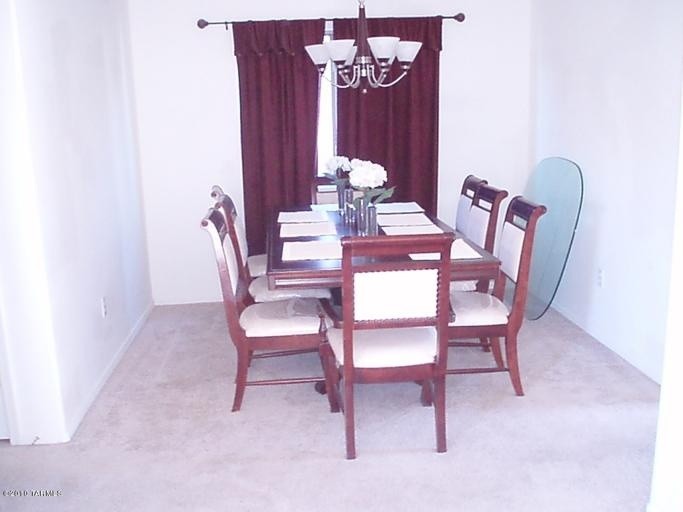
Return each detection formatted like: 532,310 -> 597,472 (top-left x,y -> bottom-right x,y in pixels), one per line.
456,176 -> 488,235
213,195 -> 331,307
450,181 -> 507,352
202,209 -> 342,413
319,232 -> 456,459
211,187 -> 267,277
445,197 -> 548,396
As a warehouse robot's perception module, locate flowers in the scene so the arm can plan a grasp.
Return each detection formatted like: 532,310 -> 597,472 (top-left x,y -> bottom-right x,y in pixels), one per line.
325,155 -> 394,208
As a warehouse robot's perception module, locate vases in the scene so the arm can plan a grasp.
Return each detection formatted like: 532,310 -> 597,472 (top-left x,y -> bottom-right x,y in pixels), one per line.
338,188 -> 377,238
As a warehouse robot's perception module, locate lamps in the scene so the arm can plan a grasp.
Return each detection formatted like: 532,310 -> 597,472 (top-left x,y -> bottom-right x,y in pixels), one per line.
303,0 -> 424,89
